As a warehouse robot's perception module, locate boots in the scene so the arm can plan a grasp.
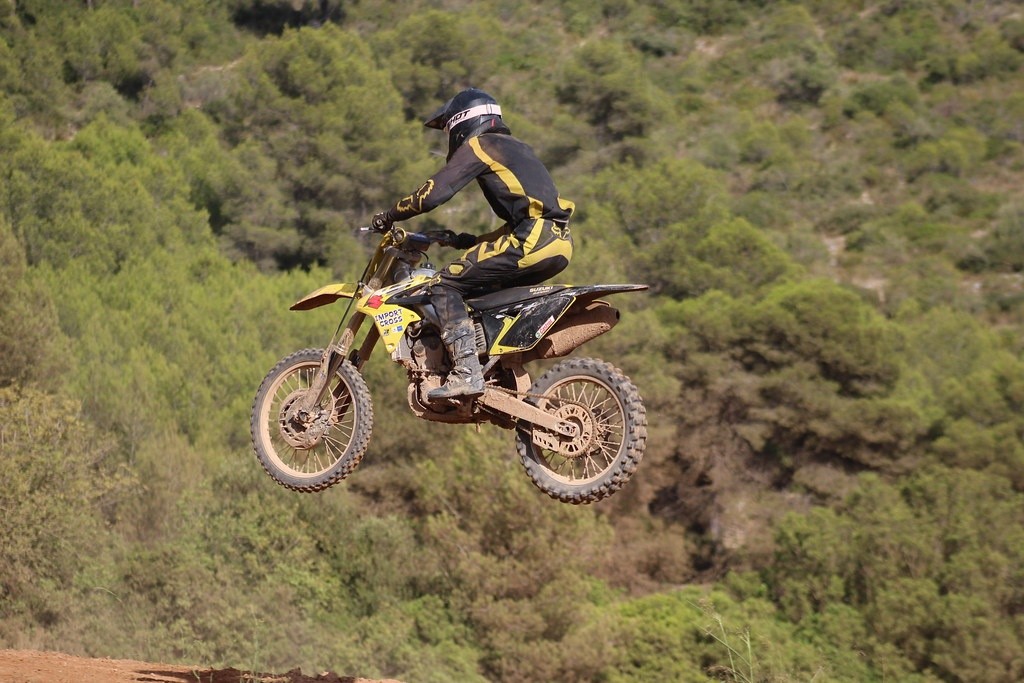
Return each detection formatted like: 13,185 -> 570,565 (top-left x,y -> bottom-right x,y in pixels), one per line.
426,317 -> 485,399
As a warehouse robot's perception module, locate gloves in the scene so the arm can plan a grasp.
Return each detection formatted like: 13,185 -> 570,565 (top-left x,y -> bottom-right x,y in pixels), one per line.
369,210 -> 394,235
456,232 -> 477,250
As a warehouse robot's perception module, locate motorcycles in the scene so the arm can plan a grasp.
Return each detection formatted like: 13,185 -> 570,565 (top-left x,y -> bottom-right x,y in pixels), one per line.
250,218 -> 650,507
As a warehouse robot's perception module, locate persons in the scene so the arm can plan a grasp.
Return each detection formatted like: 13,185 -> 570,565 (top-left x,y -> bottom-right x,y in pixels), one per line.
372,87 -> 576,400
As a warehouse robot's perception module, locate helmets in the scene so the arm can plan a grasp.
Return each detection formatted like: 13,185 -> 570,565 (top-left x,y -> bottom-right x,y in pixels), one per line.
424,90 -> 511,163
